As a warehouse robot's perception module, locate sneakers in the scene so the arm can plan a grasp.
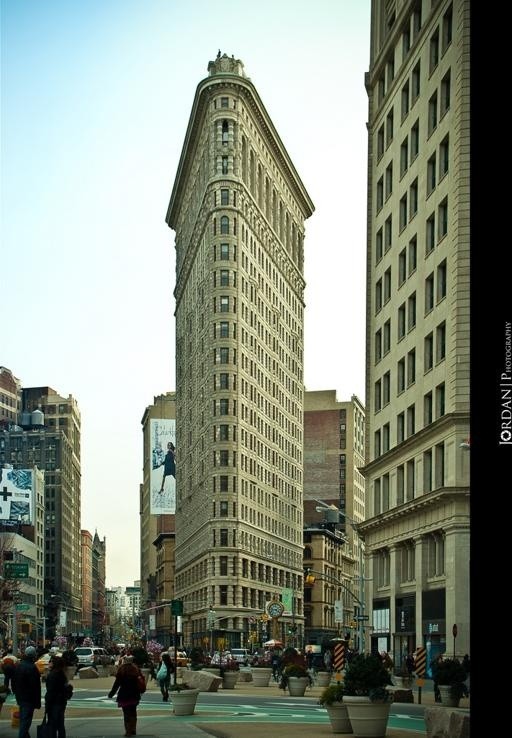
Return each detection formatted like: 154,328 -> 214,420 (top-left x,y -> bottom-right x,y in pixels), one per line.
163,693 -> 168,702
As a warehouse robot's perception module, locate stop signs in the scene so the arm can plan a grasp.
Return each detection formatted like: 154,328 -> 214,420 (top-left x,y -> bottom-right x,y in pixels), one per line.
453,622 -> 457,637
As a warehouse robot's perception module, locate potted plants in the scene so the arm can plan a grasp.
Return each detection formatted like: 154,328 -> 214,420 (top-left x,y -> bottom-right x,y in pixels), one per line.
170,681 -> 200,716
279,660 -> 313,698
220,655 -> 241,670
317,647 -> 397,738
250,648 -> 273,667
432,655 -> 467,707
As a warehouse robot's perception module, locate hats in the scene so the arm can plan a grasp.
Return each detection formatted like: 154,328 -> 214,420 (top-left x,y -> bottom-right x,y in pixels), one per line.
25,646 -> 36,656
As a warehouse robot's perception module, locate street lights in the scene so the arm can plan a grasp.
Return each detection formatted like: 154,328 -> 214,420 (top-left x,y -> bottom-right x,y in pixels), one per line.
207,606 -> 217,651
316,506 -> 364,652
50,593 -> 84,637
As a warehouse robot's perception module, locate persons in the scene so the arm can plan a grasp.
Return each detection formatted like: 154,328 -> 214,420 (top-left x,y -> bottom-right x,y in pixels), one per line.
157,442 -> 176,493
0,646 -> 470,737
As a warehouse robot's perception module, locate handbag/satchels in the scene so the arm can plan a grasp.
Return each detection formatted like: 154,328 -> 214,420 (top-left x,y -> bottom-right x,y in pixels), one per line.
137,676 -> 146,694
37,725 -> 57,738
157,665 -> 167,680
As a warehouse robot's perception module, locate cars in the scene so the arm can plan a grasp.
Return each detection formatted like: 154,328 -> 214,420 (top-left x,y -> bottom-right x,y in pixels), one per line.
36,636 -> 273,672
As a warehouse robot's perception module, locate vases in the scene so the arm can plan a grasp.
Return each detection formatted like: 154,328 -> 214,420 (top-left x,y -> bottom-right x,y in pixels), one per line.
250,667 -> 272,686
223,671 -> 238,688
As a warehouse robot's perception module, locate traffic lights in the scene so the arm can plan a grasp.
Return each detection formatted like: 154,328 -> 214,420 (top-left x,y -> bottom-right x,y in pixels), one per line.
308,573 -> 316,583
288,625 -> 292,633
21,623 -> 33,634
297,597 -> 303,615
302,566 -> 310,583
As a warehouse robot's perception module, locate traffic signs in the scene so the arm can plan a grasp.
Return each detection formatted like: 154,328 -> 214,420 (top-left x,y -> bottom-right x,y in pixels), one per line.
352,615 -> 369,622
334,599 -> 344,623
8,589 -> 22,599
4,562 -> 29,580
16,604 -> 31,610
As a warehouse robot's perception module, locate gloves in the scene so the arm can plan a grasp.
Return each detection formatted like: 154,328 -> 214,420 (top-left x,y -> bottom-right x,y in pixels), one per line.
108,691 -> 114,698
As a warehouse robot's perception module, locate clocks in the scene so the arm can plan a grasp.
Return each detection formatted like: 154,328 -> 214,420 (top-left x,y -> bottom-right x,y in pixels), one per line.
268,601 -> 284,618
